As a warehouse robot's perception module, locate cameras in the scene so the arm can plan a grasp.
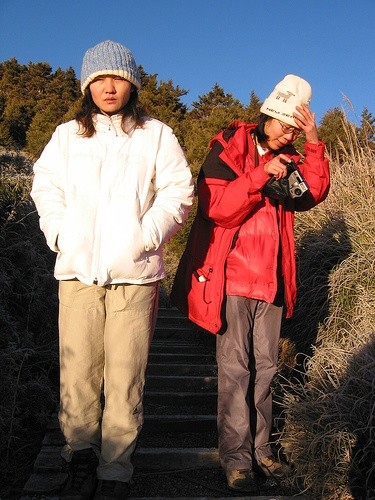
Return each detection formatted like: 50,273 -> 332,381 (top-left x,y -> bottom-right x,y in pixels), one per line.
284,160 -> 308,199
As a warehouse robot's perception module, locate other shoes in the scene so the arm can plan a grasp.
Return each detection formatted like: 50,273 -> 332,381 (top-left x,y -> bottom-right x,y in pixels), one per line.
222,455 -> 291,492
59,467 -> 130,500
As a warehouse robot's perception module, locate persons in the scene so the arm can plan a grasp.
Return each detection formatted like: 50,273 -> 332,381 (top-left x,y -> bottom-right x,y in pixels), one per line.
169,74 -> 330,493
30,40 -> 195,500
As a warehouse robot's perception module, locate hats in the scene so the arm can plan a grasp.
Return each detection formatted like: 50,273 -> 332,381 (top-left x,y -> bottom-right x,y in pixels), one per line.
259,73 -> 312,129
80,40 -> 141,96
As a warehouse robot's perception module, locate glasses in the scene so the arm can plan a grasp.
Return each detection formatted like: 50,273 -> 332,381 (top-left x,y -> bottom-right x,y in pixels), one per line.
278,120 -> 303,137
